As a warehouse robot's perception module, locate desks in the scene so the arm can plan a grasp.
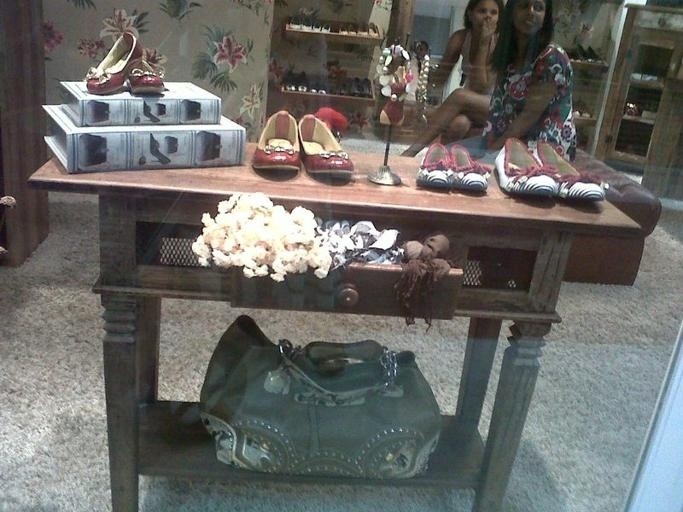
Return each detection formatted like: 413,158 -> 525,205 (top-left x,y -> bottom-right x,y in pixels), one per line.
27,144 -> 645,512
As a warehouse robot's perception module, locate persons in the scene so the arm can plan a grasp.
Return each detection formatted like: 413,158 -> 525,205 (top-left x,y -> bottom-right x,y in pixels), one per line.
398,1 -> 502,156
444,1 -> 578,169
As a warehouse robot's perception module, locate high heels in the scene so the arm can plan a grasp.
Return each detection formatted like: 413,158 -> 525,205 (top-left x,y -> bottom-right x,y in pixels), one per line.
149,134 -> 171,165
143,102 -> 159,122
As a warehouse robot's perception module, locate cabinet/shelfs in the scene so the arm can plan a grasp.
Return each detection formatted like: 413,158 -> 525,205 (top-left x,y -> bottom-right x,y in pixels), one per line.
570,60 -> 608,127
279,20 -> 383,106
603,9 -> 683,170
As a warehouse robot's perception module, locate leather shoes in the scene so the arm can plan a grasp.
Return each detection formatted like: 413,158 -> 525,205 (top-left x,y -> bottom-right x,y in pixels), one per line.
88,33 -> 142,93
287,15 -> 380,37
572,102 -> 589,116
285,73 -> 371,98
125,48 -> 164,94
298,113 -> 354,176
531,142 -> 606,201
253,110 -> 302,171
450,144 -> 488,191
567,46 -> 604,63
495,139 -> 558,200
418,143 -> 451,190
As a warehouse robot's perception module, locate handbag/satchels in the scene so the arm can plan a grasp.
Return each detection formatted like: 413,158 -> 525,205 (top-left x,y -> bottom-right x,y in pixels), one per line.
198,315 -> 442,478
